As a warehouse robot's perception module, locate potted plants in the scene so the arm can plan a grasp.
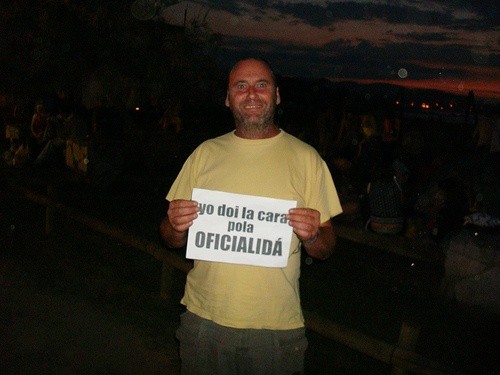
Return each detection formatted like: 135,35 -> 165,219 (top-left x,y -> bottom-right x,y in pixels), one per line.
367,182 -> 405,236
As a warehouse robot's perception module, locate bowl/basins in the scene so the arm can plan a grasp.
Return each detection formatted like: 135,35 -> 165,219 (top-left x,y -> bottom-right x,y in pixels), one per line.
370,212 -> 403,236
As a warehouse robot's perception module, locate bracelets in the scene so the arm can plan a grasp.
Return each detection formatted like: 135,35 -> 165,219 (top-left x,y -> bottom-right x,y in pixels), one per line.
300,233 -> 319,245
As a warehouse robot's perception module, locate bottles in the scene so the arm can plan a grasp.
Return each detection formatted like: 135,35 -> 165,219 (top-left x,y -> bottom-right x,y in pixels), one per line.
370,169 -> 403,218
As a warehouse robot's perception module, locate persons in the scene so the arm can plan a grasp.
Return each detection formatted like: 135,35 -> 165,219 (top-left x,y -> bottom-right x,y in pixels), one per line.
160,58 -> 344,375
0,72 -> 499,240
